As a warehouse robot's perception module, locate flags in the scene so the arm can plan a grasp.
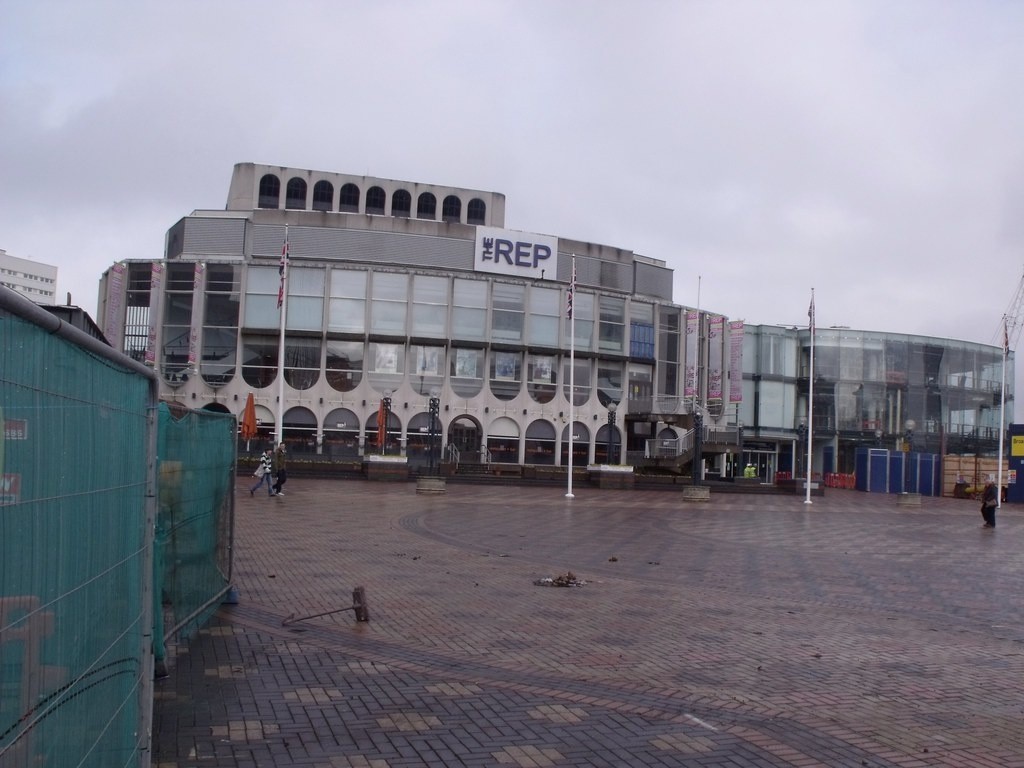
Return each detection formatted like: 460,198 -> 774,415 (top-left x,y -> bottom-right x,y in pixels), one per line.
567,259 -> 577,319
1005,322 -> 1009,357
808,298 -> 815,335
277,235 -> 290,308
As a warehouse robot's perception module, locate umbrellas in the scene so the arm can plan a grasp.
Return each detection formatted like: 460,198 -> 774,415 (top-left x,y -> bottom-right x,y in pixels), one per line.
376,399 -> 389,455
241,392 -> 258,452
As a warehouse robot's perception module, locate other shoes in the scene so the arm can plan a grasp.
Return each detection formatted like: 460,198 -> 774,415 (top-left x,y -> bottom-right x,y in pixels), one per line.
277,492 -> 284,496
251,490 -> 254,496
270,493 -> 277,496
984,523 -> 995,528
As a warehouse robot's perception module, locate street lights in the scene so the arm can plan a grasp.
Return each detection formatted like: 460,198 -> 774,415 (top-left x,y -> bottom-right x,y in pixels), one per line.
798,416 -> 806,478
905,420 -> 914,492
608,403 -> 616,465
382,390 -> 391,456
875,430 -> 882,449
693,412 -> 702,486
737,420 -> 744,476
428,387 -> 441,476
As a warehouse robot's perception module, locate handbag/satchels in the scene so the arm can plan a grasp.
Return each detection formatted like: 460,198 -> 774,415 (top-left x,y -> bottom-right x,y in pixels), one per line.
986,500 -> 997,508
254,464 -> 264,478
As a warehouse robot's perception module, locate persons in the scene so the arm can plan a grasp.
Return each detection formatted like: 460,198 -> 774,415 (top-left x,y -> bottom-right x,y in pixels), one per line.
981,474 -> 999,528
744,463 -> 758,478
273,442 -> 287,496
250,447 -> 277,497
